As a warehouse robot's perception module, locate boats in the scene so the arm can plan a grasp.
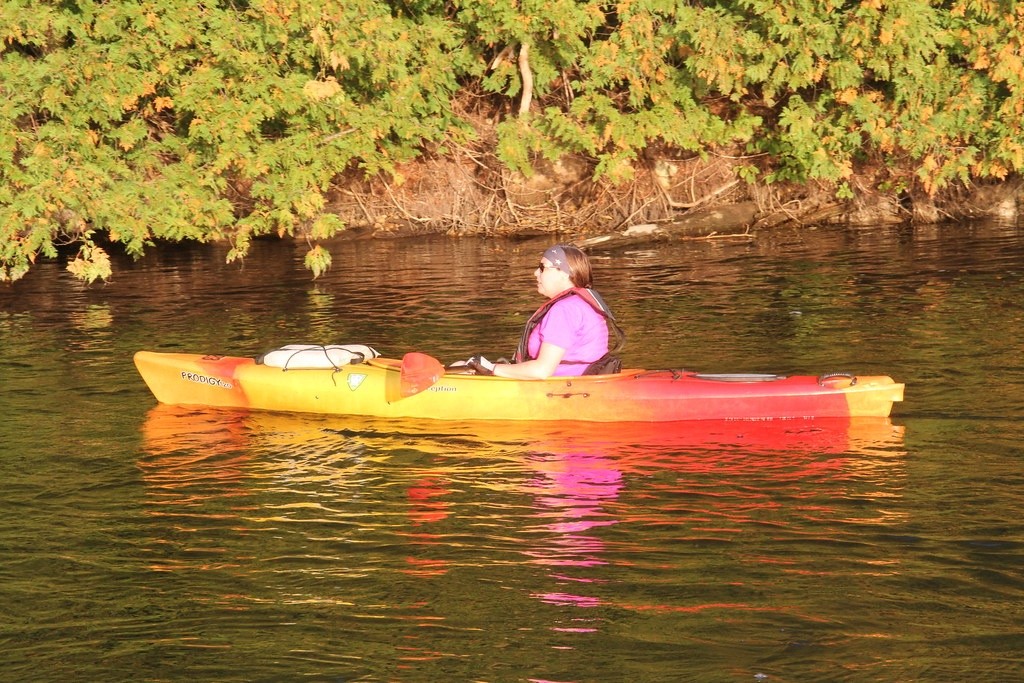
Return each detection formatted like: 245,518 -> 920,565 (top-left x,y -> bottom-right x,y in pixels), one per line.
132,350 -> 905,432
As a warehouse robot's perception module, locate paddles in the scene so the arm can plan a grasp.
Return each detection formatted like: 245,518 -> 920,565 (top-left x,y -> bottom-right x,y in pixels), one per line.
398,352 -> 516,399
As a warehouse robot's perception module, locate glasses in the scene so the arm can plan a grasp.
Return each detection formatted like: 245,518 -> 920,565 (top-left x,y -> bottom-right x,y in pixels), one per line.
539,262 -> 560,273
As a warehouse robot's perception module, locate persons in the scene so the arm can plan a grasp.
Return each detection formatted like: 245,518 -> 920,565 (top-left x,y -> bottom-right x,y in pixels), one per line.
446,244 -> 620,381
